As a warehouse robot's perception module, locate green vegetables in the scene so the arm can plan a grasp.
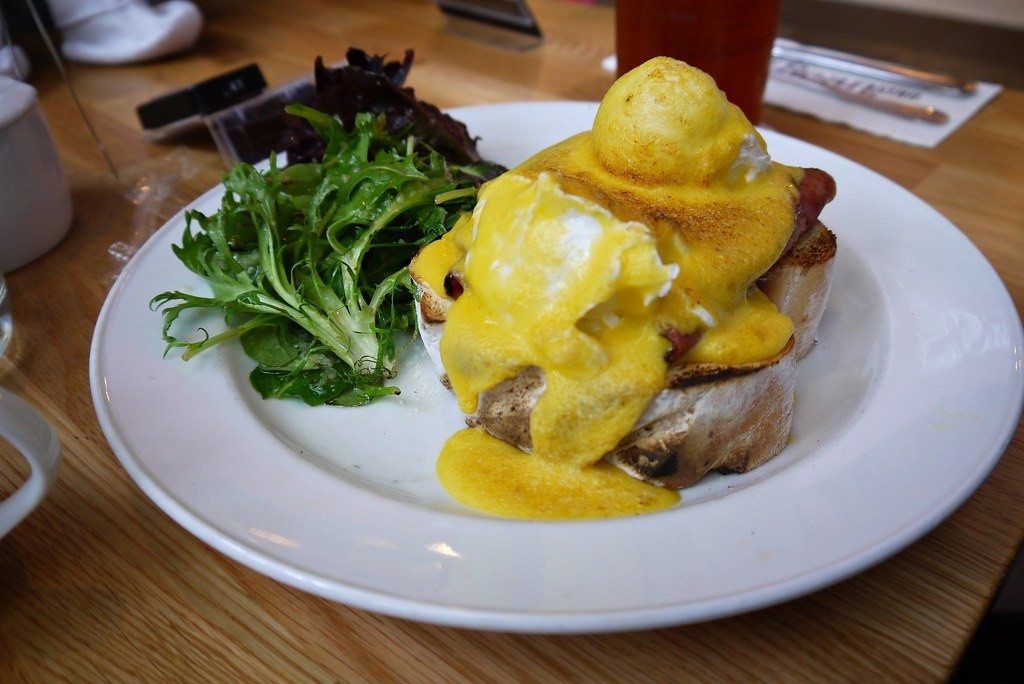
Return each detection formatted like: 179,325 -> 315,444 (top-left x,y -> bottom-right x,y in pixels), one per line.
149,45 -> 513,409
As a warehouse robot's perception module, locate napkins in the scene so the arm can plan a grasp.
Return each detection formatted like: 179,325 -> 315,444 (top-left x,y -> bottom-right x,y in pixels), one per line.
601,37 -> 1003,148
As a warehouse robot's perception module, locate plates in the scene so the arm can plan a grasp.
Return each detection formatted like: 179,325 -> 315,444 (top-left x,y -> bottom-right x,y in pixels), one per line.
87,100 -> 1024,633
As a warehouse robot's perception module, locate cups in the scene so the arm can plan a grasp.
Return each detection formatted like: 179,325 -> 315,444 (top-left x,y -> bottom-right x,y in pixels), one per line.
0,273 -> 14,355
614,1 -> 781,124
